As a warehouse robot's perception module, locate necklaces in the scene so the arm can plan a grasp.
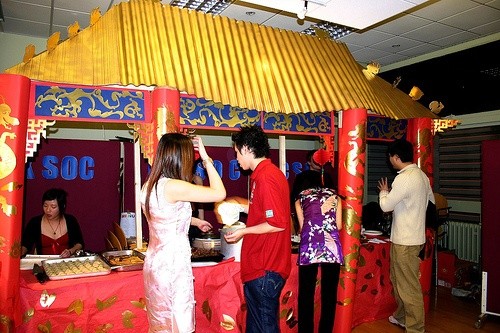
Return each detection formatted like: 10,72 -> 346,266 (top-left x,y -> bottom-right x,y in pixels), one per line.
46,216 -> 60,235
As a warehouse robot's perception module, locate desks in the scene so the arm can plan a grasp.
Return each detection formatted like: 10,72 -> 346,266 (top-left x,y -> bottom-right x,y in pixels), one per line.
15,228 -> 434,333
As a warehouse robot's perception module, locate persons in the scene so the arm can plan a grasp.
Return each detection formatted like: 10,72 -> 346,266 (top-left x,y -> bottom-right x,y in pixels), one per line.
224,125 -> 292,333
293,171 -> 345,333
20,189 -> 84,259
189,173 -> 205,238
377,140 -> 436,333
141,133 -> 227,333
293,150 -> 333,200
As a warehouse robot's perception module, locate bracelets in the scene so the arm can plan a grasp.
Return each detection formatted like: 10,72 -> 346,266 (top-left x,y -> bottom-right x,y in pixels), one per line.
202,157 -> 214,168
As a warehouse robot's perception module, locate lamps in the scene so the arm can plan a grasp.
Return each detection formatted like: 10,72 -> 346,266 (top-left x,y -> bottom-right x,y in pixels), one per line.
297,1 -> 308,19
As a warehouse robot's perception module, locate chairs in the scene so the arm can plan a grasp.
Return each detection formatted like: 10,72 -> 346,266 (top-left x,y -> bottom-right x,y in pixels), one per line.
434,193 -> 452,252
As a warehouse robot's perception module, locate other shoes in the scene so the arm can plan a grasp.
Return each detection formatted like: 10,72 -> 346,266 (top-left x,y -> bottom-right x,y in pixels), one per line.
389,316 -> 405,328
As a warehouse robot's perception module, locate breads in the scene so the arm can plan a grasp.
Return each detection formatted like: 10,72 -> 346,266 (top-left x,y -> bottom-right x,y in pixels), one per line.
44,259 -> 108,277
106,256 -> 143,265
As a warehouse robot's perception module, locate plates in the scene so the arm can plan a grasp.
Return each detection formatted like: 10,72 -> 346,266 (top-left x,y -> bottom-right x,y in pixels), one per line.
364,230 -> 383,236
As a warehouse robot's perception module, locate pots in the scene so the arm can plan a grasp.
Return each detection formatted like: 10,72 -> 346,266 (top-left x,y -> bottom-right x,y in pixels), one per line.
193,231 -> 221,251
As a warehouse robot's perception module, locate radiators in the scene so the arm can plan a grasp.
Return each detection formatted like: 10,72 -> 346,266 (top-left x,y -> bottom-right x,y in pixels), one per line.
441,222 -> 482,264
